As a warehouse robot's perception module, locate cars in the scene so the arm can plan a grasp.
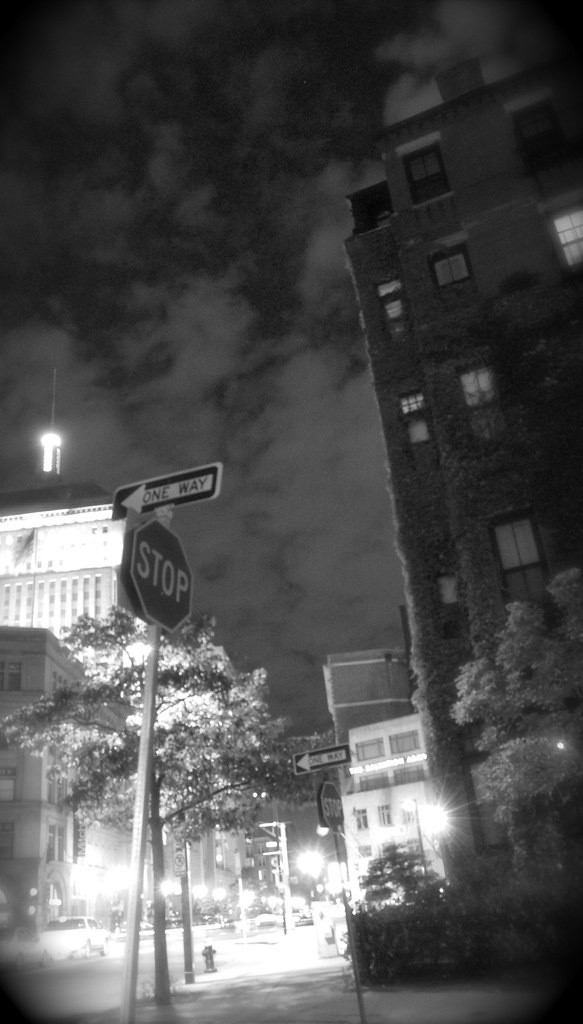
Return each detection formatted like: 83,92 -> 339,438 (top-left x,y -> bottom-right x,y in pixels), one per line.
2,927 -> 50,973
40,917 -> 113,960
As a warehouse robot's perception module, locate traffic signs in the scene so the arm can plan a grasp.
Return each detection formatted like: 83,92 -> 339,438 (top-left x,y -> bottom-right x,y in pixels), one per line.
109,462 -> 221,523
292,744 -> 347,774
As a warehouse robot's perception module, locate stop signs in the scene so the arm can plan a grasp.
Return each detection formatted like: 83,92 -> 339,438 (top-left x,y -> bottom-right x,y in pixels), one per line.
317,782 -> 345,833
119,517 -> 193,634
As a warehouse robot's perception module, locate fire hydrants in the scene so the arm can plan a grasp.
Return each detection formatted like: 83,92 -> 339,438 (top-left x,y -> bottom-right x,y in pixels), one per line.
201,946 -> 217,973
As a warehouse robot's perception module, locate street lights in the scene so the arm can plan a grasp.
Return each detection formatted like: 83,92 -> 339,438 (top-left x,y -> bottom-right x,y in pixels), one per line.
403,798 -> 427,876
